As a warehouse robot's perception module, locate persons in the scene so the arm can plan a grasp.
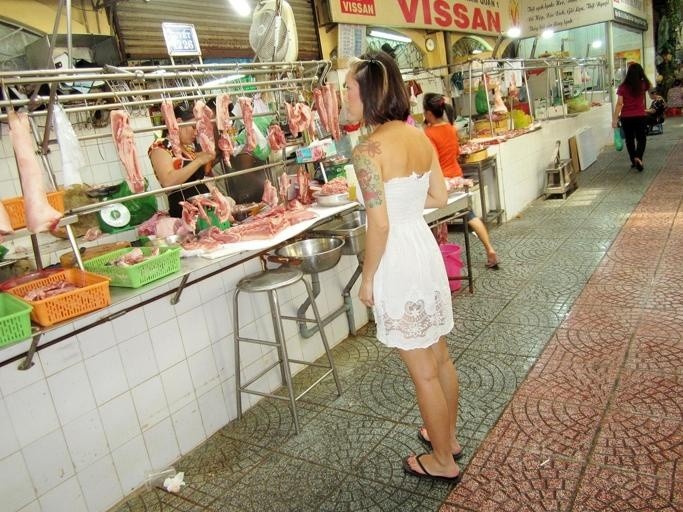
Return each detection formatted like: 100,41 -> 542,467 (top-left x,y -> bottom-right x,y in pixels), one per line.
612,64 -> 652,172
342,49 -> 463,482
148,103 -> 213,218
644,88 -> 665,136
667,81 -> 683,108
422,94 -> 496,268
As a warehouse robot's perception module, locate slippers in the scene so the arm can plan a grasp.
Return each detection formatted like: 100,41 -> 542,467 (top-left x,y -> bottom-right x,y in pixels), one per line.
402,453 -> 463,483
417,430 -> 464,459
484,260 -> 501,269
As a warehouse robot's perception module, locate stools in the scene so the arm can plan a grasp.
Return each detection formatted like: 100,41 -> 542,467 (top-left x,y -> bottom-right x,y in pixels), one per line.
667,108 -> 681,117
542,158 -> 579,199
649,123 -> 663,135
233,268 -> 344,437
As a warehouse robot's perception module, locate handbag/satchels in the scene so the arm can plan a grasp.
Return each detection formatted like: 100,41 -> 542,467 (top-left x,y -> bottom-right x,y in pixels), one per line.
613,127 -> 623,151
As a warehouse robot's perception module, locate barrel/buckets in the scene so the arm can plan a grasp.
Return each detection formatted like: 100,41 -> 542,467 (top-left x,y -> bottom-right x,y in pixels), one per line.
438,244 -> 465,292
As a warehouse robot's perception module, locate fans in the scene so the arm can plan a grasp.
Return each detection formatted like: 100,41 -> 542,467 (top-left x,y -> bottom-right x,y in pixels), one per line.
248,0 -> 299,68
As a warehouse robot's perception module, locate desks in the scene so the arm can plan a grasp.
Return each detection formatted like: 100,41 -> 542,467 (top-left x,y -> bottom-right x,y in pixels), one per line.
422,192 -> 474,295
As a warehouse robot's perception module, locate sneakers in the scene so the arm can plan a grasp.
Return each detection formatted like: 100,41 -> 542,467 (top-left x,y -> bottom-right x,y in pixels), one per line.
634,157 -> 644,172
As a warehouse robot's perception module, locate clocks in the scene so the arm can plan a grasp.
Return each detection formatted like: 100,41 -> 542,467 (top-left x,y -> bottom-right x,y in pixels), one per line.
425,38 -> 435,51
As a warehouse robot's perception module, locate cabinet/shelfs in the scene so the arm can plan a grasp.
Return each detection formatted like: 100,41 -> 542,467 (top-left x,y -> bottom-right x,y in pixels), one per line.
444,154 -> 505,233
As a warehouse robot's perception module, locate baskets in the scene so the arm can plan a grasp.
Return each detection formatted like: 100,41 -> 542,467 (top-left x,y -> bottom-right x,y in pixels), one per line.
0,267 -> 112,347
1,190 -> 64,230
82,246 -> 182,289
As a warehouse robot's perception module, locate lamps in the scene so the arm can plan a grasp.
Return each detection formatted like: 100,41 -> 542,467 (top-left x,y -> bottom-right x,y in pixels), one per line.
366,26 -> 412,43
529,28 -> 553,59
491,26 -> 521,60
585,39 -> 601,58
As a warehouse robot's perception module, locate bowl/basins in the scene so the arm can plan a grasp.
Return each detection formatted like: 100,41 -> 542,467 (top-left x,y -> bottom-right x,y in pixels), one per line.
312,190 -> 354,207
274,237 -> 346,274
